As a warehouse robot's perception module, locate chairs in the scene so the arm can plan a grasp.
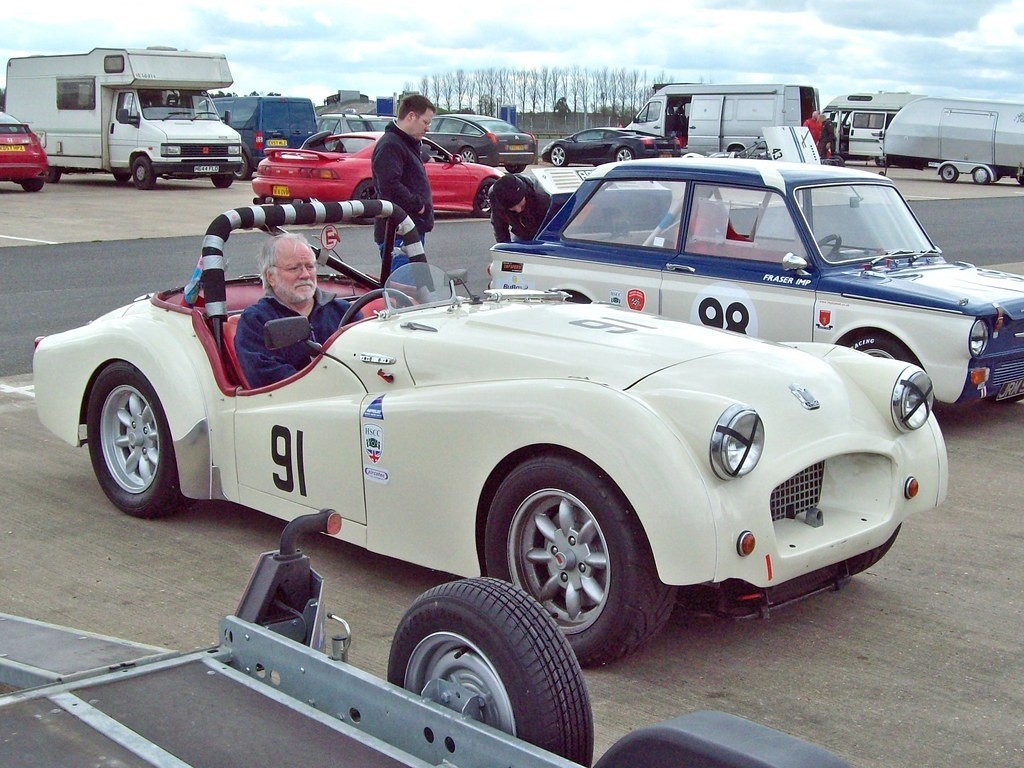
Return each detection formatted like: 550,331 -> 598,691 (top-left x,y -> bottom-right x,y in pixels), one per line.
691,198 -> 730,257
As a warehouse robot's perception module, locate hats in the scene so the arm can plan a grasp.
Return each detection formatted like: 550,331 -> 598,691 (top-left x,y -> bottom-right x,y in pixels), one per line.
493,174 -> 525,210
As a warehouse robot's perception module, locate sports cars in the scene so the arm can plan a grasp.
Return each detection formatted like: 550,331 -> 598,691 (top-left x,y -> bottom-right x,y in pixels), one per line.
541,127 -> 680,169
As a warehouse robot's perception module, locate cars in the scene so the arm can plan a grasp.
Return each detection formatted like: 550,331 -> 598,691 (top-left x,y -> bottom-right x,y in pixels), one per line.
250,130 -> 503,218
318,113 -> 399,150
486,156 -> 1023,422
679,126 -> 841,167
421,115 -> 539,172
0,111 -> 50,192
32,200 -> 950,667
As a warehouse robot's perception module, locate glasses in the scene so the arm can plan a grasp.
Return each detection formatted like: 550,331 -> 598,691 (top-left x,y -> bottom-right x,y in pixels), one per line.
415,112 -> 431,127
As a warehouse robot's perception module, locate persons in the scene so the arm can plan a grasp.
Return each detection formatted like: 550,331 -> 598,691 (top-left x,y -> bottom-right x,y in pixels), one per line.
822,114 -> 837,154
234,233 -> 363,389
374,95 -> 437,286
488,174 -> 551,242
803,111 -> 822,148
855,115 -> 865,127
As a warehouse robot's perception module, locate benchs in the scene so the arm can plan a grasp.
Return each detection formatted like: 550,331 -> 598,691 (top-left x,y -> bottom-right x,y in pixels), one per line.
221,295 -> 420,390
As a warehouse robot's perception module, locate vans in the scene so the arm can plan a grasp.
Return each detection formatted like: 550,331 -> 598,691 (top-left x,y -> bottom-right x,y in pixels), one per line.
625,82 -> 820,159
198,97 -> 319,181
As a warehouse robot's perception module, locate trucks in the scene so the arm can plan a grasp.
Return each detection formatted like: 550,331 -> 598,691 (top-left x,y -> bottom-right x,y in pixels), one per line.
1,511 -> 864,768
822,91 -> 929,166
3,47 -> 244,188
